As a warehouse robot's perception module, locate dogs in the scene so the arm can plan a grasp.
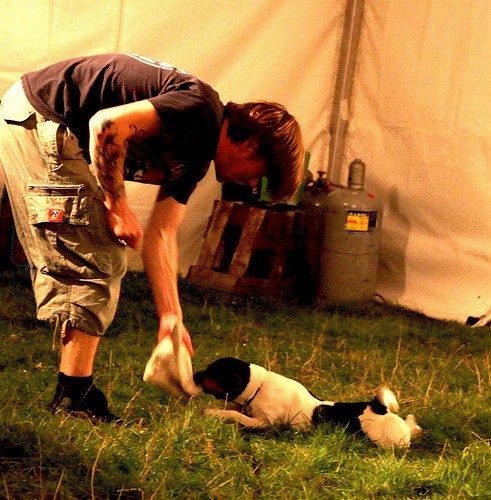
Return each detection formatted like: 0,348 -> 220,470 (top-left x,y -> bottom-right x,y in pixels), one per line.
191,356 -> 422,452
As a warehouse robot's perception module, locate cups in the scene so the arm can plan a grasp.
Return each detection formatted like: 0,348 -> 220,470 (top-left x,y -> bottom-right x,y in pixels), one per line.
124,181 -> 161,232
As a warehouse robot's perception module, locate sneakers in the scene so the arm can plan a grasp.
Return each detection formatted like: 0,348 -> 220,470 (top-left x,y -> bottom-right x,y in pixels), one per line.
50,386 -> 122,425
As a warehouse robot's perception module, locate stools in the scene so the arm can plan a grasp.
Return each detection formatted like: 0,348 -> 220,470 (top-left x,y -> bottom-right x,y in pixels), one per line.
185,197 -> 303,308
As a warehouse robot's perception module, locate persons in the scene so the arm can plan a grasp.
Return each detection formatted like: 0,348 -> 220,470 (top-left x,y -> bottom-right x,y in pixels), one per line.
2,52 -> 305,427
